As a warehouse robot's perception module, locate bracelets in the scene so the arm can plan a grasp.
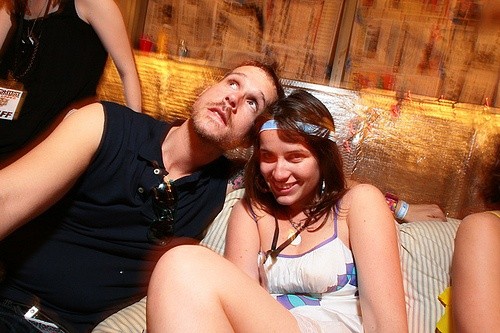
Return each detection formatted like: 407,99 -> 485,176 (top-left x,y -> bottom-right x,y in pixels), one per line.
385,192 -> 399,214
396,201 -> 408,220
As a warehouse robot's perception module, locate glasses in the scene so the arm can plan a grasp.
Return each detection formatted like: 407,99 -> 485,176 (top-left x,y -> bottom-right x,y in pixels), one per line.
146,179 -> 176,245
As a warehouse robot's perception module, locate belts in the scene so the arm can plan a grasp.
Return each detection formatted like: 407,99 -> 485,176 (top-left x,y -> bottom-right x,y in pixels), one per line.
0,298 -> 64,333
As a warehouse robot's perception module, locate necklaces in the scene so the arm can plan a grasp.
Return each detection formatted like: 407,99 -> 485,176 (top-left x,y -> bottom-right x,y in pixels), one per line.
285,209 -> 302,246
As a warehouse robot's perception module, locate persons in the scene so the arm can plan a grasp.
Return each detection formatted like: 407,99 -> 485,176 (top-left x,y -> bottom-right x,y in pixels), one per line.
0,60 -> 444,333
0,0 -> 142,164
437,211 -> 500,333
148,89 -> 408,333
